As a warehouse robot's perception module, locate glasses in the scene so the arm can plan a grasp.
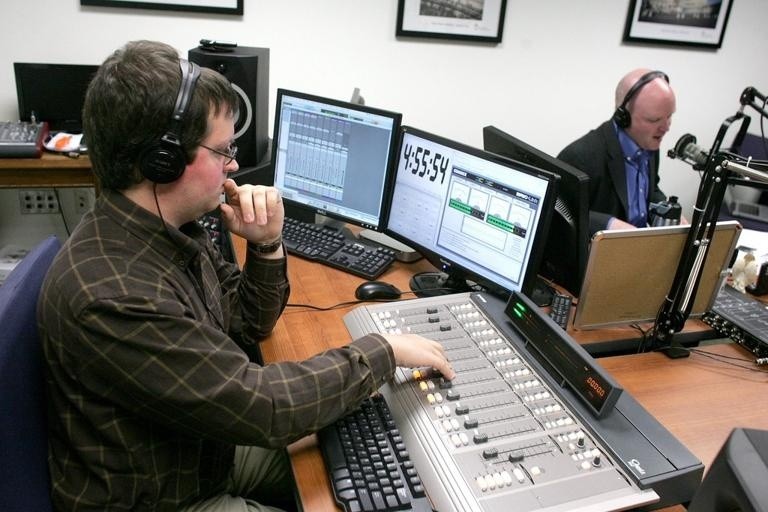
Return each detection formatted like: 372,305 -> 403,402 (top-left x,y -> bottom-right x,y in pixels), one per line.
184,135 -> 239,165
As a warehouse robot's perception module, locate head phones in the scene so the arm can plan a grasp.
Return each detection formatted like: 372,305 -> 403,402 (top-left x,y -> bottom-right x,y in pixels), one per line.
613,70 -> 669,128
139,59 -> 200,182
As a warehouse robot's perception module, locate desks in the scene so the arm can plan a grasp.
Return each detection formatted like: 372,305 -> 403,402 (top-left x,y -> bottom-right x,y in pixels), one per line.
0,123 -> 194,248
574,343 -> 768,512
535,277 -> 768,357
217,203 -> 690,512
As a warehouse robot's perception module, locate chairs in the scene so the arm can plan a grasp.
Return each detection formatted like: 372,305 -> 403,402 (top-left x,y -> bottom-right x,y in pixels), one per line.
0,237 -> 88,512
720,130 -> 768,230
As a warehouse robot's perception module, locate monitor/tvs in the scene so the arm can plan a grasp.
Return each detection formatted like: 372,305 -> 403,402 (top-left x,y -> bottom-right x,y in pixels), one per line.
482,125 -> 591,301
13,62 -> 100,132
380,125 -> 559,296
269,88 -> 402,234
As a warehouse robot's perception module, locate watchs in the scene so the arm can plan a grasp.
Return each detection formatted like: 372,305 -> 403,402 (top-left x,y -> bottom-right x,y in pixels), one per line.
247,237 -> 284,253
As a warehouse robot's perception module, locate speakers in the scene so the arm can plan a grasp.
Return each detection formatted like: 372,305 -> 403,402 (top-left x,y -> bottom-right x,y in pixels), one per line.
687,428 -> 767,511
187,46 -> 269,165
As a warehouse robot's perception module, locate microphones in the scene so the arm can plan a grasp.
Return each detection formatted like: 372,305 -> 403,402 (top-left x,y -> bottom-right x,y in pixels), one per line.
668,133 -> 707,163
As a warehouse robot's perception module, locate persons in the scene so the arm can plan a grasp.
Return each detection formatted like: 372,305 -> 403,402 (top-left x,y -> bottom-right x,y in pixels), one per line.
34,40 -> 456,510
543,67 -> 692,306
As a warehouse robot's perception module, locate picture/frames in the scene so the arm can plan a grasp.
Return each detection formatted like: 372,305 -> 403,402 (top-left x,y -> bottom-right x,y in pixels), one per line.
395,0 -> 507,43
623,0 -> 733,50
81,0 -> 244,14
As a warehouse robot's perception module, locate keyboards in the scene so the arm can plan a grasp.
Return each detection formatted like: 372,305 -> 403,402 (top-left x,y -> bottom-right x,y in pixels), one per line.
316,392 -> 433,511
282,215 -> 395,279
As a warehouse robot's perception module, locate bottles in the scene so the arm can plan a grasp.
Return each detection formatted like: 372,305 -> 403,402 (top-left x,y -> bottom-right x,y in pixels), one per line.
650,195 -> 681,227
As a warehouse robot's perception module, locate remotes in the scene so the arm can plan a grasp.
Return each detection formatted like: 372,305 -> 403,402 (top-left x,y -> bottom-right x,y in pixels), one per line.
549,296 -> 572,331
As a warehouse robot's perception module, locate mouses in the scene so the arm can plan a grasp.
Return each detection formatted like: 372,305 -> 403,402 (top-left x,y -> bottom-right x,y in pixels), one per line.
355,281 -> 399,301
